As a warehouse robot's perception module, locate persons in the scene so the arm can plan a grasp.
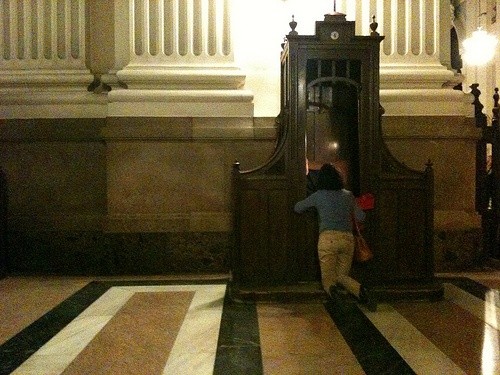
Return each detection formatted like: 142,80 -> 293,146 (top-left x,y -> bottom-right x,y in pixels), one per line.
291,162 -> 377,315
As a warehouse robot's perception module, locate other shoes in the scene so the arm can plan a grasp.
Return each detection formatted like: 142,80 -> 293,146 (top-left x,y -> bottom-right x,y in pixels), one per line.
330,285 -> 351,313
364,281 -> 376,311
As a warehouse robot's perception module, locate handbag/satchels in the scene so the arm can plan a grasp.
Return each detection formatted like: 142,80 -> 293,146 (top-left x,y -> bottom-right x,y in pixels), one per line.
355,233 -> 373,261
